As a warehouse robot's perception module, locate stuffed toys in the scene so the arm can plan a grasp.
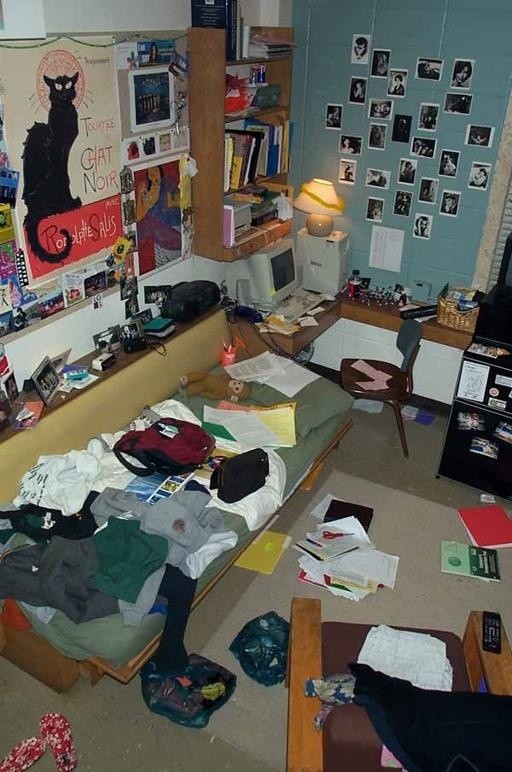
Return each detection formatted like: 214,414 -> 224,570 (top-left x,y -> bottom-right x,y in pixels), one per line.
179,368 -> 253,404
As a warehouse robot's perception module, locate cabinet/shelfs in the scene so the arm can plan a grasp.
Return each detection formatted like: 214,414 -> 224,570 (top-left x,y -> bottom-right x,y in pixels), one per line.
434,334 -> 511,502
185,23 -> 293,260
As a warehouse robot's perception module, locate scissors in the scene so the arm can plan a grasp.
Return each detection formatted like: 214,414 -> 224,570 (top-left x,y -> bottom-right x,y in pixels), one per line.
323,531 -> 356,539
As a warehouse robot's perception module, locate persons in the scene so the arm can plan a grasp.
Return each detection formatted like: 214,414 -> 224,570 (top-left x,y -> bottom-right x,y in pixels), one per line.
136,166 -> 181,229
325,34 -> 496,240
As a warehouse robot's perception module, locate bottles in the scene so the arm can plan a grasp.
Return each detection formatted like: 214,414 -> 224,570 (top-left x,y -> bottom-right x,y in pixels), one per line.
348,269 -> 363,300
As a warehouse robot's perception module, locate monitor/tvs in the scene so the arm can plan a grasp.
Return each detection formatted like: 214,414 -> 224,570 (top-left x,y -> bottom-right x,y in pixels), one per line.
235,239 -> 299,311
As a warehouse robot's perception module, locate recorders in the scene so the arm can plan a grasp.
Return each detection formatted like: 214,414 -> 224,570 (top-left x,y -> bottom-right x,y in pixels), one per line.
161,280 -> 220,323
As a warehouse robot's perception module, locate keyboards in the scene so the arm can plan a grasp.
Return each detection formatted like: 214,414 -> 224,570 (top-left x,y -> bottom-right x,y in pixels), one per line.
275,287 -> 324,325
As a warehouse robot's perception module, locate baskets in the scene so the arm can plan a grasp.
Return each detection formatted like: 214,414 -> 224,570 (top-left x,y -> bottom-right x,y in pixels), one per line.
436,285 -> 479,335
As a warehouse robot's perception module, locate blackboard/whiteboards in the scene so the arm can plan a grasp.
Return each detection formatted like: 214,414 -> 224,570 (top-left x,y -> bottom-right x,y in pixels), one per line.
0,34 -> 125,290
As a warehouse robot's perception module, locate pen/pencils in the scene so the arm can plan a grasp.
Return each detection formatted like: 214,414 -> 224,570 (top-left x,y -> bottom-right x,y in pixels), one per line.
221,335 -> 246,354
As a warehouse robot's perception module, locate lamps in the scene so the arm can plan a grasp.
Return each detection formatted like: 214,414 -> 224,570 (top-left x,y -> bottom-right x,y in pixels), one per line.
293,178 -> 342,236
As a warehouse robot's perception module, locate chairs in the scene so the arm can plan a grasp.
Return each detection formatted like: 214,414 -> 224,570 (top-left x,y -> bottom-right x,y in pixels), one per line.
340,318 -> 423,458
281,590 -> 511,771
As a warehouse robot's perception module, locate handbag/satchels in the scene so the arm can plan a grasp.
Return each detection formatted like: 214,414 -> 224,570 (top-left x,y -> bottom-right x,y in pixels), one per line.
211,450 -> 269,502
112,417 -> 217,477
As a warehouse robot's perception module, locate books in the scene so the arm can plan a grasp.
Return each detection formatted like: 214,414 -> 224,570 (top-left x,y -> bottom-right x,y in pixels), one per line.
439,540 -> 502,582
457,503 -> 512,549
191,0 -> 299,65
223,205 -> 236,249
217,115 -> 293,191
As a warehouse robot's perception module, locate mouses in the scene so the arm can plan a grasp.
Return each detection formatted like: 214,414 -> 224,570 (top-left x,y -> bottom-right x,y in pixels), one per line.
320,293 -> 336,301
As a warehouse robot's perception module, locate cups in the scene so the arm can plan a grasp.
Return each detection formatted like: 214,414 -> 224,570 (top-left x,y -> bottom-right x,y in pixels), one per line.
221,347 -> 237,367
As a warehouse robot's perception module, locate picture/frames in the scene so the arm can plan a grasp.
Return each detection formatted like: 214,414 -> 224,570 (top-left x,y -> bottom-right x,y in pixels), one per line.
29,356 -> 64,407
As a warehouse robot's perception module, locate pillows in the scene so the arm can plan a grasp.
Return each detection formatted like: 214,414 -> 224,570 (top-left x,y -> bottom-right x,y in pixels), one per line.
249,366 -> 353,440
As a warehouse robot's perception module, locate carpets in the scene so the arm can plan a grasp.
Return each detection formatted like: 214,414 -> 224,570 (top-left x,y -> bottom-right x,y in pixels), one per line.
202,469 -> 512,771
